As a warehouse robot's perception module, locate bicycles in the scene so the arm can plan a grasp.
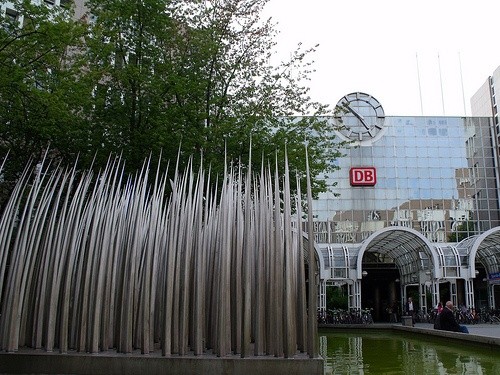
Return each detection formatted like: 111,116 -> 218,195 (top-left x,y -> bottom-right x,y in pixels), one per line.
418,307 -> 500,323
320,309 -> 373,326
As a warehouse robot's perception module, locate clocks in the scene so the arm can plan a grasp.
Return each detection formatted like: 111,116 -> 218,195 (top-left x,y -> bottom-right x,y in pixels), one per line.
332,92 -> 385,141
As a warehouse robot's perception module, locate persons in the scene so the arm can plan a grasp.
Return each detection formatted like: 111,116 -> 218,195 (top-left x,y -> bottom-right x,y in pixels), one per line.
441,301 -> 470,334
406,297 -> 416,327
437,302 -> 443,315
